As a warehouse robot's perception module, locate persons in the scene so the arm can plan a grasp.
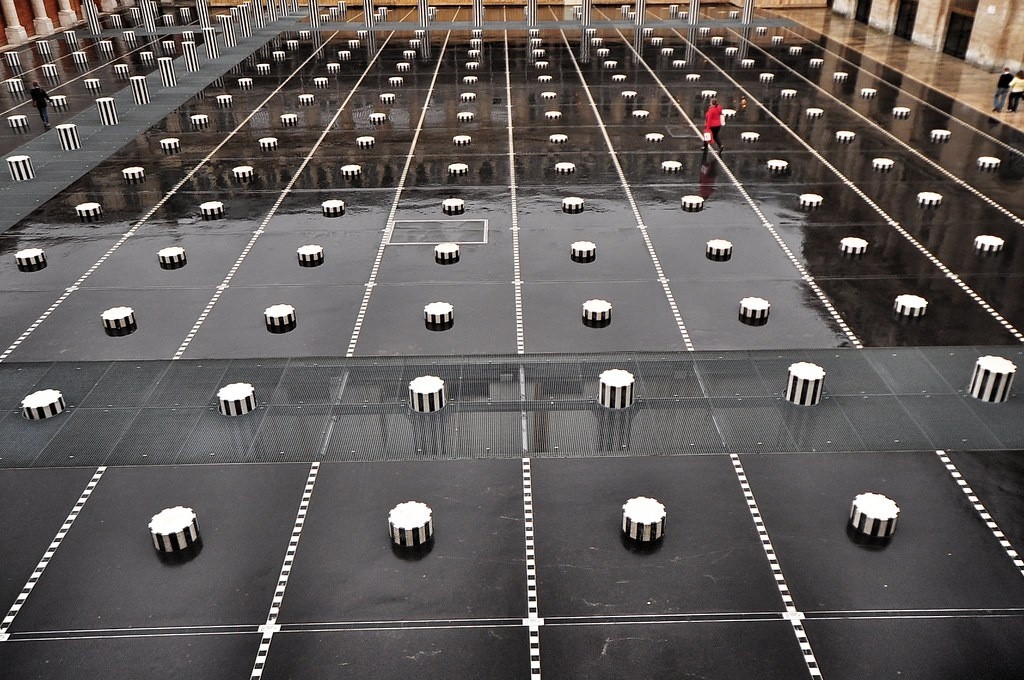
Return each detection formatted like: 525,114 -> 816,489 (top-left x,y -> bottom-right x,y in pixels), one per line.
701,99 -> 724,154
1006,70 -> 1024,113
30,82 -> 52,127
992,67 -> 1014,113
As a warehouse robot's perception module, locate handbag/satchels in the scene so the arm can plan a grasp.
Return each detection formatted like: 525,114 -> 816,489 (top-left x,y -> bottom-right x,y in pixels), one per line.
704,130 -> 714,144
33,100 -> 37,107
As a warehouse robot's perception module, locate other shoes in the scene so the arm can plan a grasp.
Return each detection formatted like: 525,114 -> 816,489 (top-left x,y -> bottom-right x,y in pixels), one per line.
46,123 -> 50,126
701,147 -> 708,150
997,110 -> 1001,112
992,108 -> 997,111
719,145 -> 724,154
